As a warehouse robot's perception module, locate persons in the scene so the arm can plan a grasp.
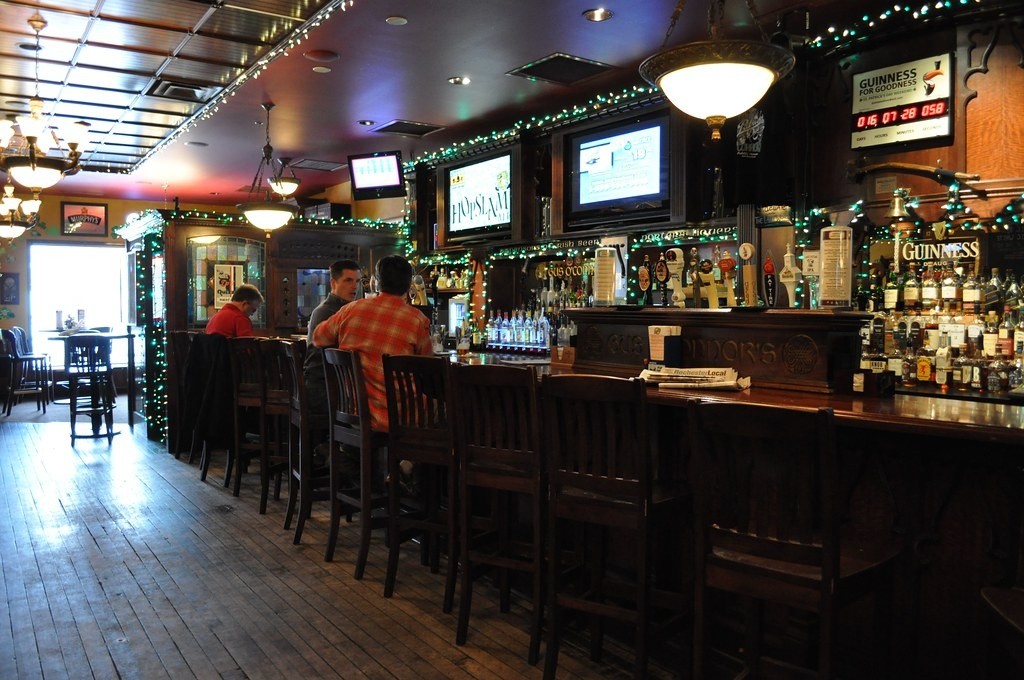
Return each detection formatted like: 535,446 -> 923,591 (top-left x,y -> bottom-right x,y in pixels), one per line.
303,260 -> 361,474
205,284 -> 264,438
312,256 -> 445,496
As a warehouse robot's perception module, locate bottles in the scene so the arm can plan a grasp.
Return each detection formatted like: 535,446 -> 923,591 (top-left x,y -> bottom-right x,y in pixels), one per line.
411,184 -> 1024,394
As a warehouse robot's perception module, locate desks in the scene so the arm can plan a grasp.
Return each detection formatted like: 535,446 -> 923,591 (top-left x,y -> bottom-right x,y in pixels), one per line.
37,328 -> 128,409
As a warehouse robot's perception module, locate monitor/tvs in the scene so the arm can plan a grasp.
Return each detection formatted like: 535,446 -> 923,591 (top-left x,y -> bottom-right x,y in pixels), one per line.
446,150 -> 512,238
571,116 -> 670,212
347,150 -> 407,200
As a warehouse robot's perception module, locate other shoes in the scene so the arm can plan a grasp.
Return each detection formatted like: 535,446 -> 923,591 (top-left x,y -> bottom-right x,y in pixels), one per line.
327,455 -> 360,477
386,463 -> 433,502
314,443 -> 341,457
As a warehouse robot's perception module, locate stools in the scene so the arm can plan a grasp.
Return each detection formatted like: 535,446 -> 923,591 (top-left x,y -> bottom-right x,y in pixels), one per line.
169,330 -> 1024,680
0,326 -> 117,446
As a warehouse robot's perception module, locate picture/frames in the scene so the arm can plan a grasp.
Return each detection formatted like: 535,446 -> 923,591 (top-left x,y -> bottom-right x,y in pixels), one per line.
59,200 -> 109,238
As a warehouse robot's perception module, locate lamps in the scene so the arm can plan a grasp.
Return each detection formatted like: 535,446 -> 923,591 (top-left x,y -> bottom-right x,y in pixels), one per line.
637,0 -> 796,140
266,158 -> 301,202
0,7 -> 91,245
236,103 -> 300,239
